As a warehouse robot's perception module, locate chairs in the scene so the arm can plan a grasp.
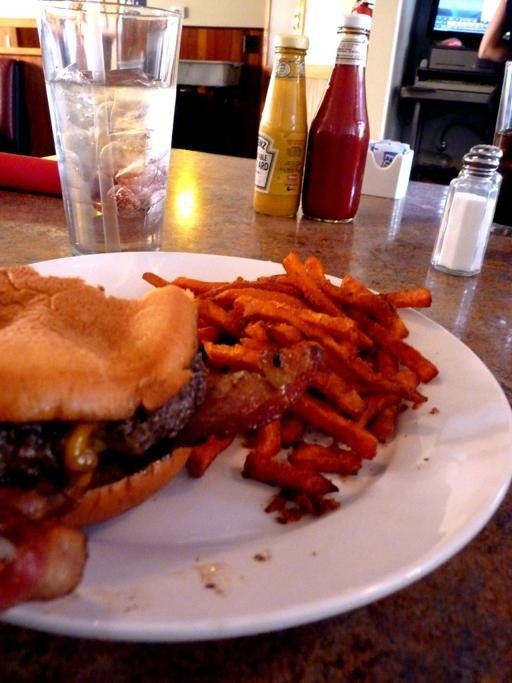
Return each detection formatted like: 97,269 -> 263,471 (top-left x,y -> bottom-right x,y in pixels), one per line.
0,56 -> 33,156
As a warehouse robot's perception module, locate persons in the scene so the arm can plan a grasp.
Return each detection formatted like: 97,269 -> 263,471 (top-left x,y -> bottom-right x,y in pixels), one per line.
477,0 -> 512,149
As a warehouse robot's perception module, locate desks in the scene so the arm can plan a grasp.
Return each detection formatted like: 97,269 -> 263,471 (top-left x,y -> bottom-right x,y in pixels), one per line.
398,77 -> 502,187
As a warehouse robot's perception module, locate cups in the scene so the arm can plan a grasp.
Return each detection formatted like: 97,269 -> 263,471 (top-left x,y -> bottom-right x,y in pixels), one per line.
35,3 -> 180,253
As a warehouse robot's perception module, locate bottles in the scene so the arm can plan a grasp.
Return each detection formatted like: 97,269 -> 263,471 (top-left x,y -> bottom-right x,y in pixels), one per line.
431,143 -> 501,278
253,33 -> 305,219
310,4 -> 372,219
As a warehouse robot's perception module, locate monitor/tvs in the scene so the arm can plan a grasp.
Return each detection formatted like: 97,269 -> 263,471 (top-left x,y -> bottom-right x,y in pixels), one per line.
426,1 -> 512,39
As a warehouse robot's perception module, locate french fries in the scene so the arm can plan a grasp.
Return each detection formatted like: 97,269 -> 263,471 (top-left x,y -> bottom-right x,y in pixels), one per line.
143,248 -> 441,525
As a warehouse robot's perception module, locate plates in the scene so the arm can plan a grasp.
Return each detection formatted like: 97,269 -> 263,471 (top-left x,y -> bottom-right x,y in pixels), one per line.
3,252 -> 511,640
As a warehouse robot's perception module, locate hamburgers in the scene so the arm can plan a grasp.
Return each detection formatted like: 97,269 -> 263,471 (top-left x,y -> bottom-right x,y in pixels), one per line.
2,262 -> 208,531
